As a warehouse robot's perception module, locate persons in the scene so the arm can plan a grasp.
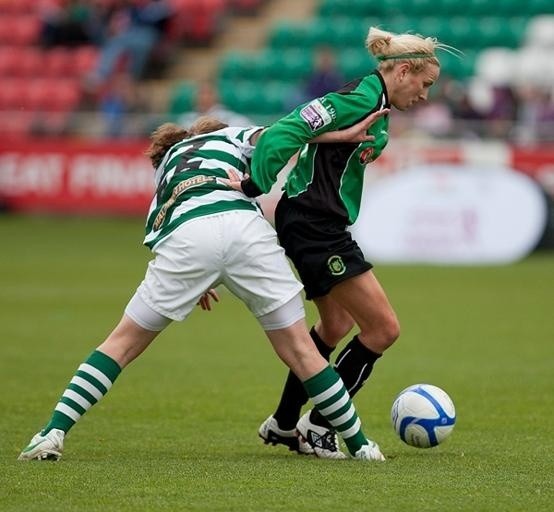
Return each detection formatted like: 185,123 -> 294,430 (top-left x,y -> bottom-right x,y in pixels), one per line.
288,45 -> 346,108
17,107 -> 394,463
32,0 -> 186,146
169,77 -> 252,131
214,26 -> 442,460
434,75 -> 552,145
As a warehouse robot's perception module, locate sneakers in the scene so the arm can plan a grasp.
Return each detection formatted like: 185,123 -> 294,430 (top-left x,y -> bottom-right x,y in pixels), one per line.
296,410 -> 345,459
17,427 -> 66,462
352,437 -> 385,462
257,415 -> 315,455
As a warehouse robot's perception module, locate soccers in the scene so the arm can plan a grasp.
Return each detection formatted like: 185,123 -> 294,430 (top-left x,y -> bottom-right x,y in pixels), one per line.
390,383 -> 456,449
